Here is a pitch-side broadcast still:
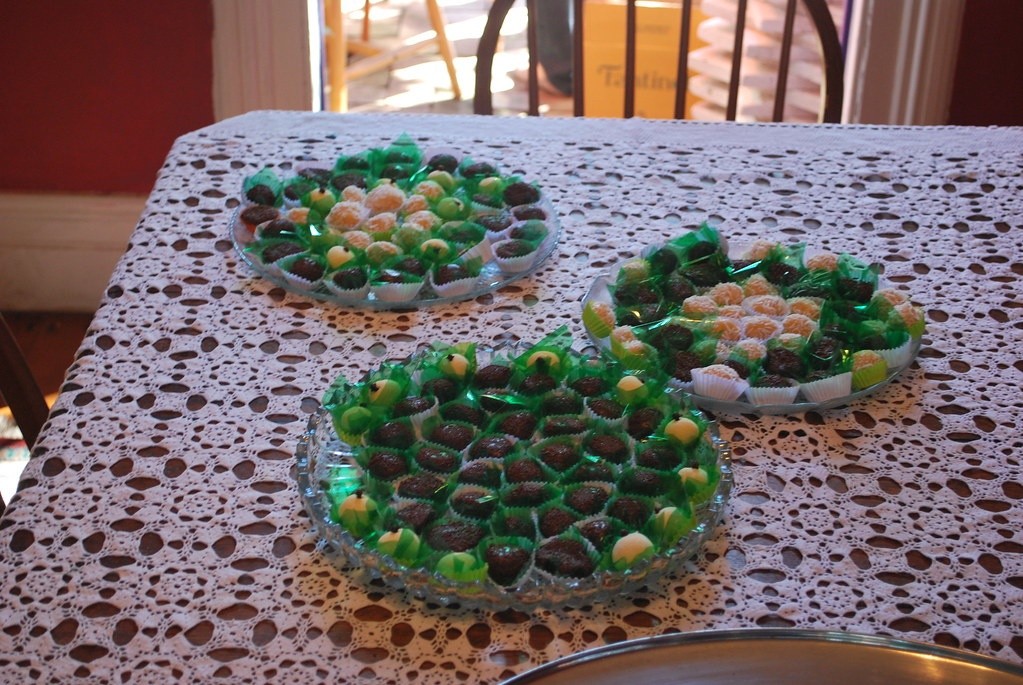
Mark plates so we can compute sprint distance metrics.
[492,626,1021,685]
[290,342,736,606]
[581,240,924,414]
[229,159,560,308]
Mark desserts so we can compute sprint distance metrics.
[583,222,927,407]
[315,321,725,593]
[238,134,553,303]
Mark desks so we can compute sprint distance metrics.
[2,108,1021,683]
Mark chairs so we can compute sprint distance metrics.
[472,0,844,122]
